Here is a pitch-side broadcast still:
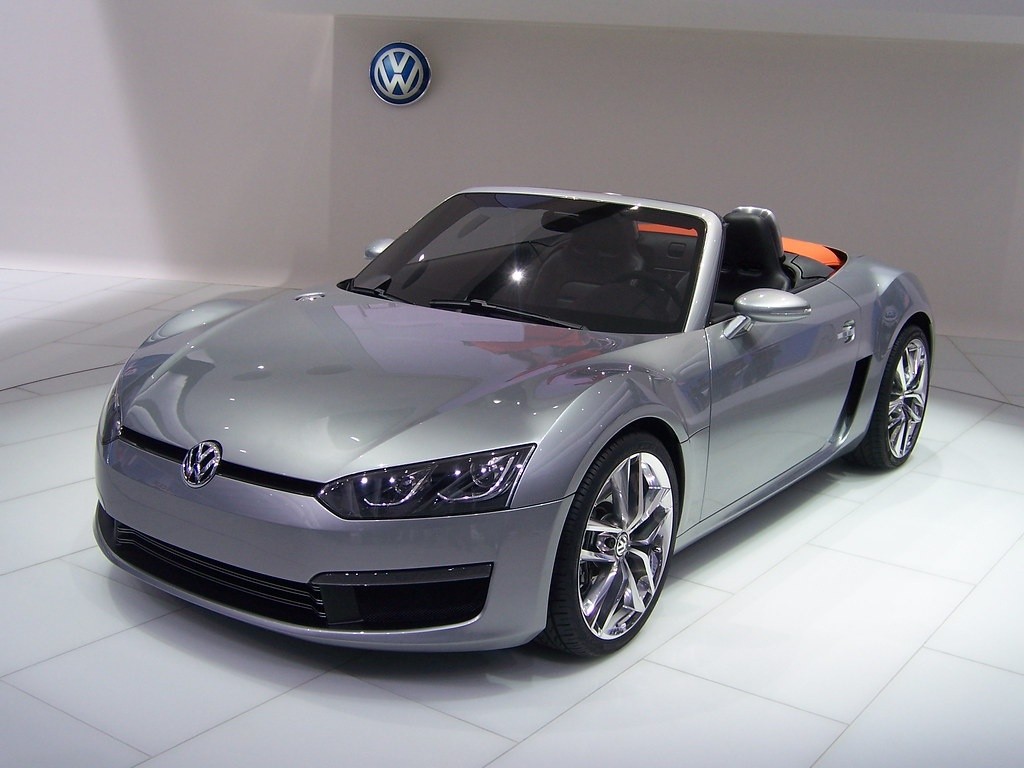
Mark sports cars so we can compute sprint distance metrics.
[93,186,935,660]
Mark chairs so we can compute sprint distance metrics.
[667,208,788,336]
[525,216,647,335]
